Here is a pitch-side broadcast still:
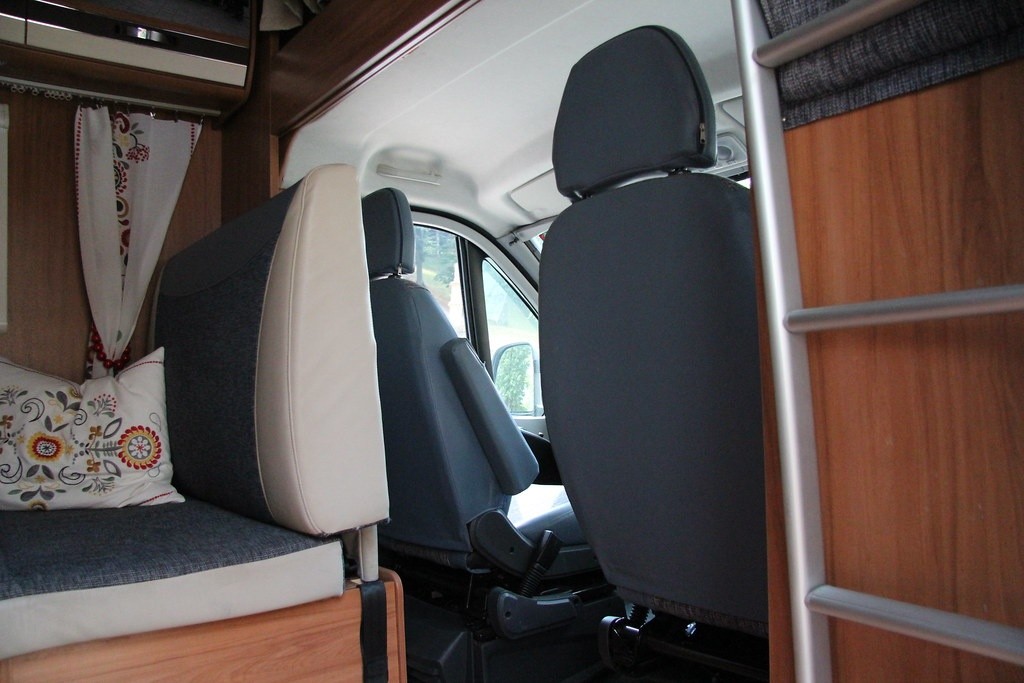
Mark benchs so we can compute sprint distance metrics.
[0,160,407,683]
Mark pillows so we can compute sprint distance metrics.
[0,345,187,510]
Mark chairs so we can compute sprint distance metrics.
[549,24,771,682]
[359,186,610,639]
